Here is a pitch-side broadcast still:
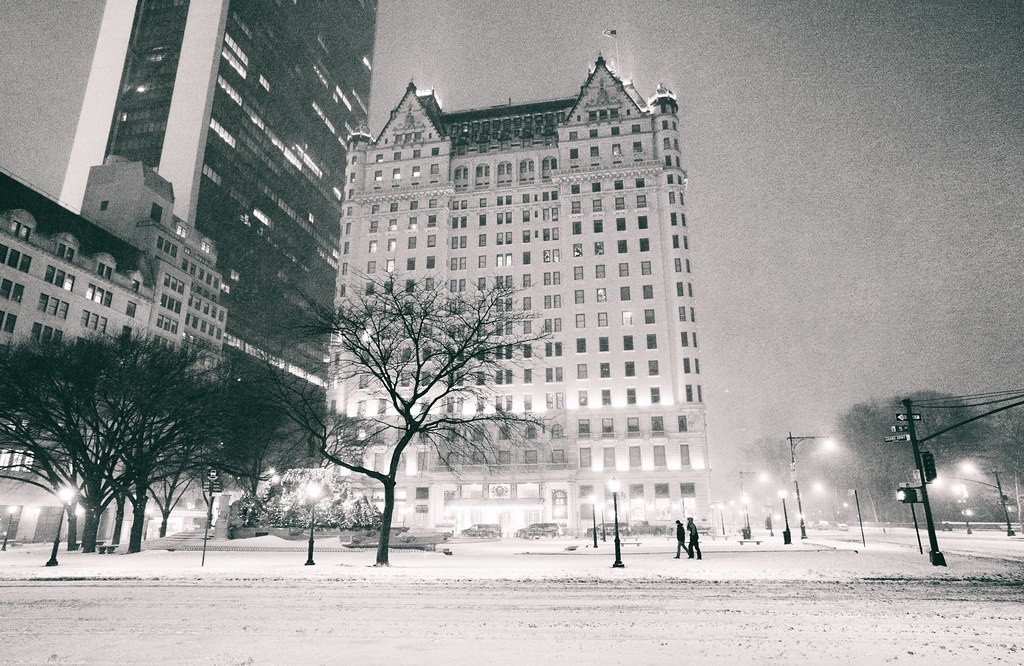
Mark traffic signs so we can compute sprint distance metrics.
[895,413,922,421]
[890,426,908,433]
[882,434,913,443]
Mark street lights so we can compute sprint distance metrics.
[142,509,153,542]
[787,433,835,539]
[303,483,321,566]
[719,502,727,536]
[608,479,625,569]
[779,490,792,539]
[739,470,768,534]
[1,505,18,552]
[45,487,74,567]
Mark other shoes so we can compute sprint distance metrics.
[674,556,680,559]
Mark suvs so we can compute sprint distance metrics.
[516,523,562,540]
[586,522,630,538]
[461,524,502,538]
[432,522,455,539]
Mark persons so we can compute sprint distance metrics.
[227,506,236,539]
[674,520,689,558]
[687,518,701,559]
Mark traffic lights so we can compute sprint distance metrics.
[922,452,938,481]
[897,489,917,503]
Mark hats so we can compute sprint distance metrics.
[687,517,693,521]
[676,520,680,523]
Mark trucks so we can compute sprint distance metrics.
[631,520,669,538]
